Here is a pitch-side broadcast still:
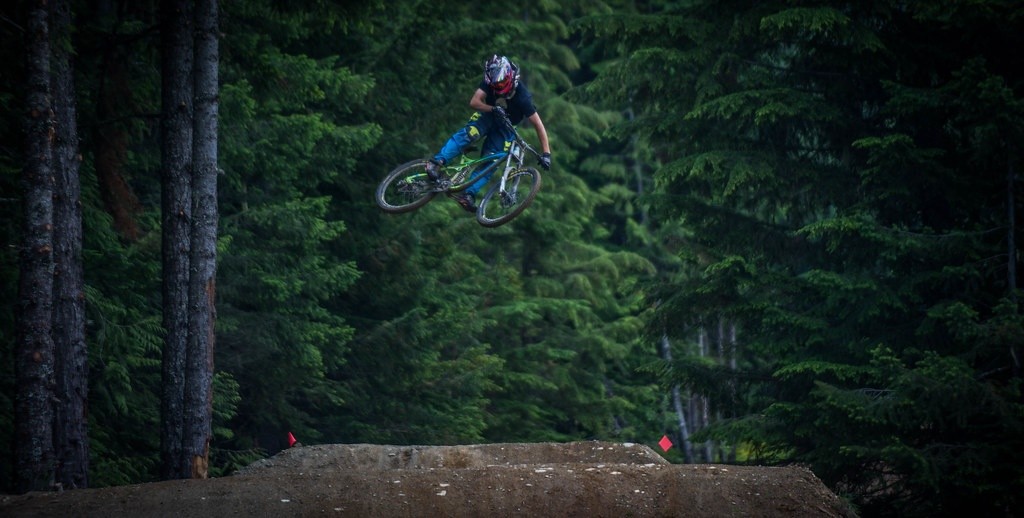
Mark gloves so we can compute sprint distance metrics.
[539,153,551,169]
[491,106,509,121]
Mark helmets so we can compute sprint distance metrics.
[484,54,521,98]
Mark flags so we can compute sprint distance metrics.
[658,435,672,452]
[289,432,296,447]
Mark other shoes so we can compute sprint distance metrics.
[461,193,478,212]
[425,159,442,180]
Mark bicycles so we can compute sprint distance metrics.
[375,108,549,226]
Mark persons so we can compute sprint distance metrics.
[425,54,550,212]
